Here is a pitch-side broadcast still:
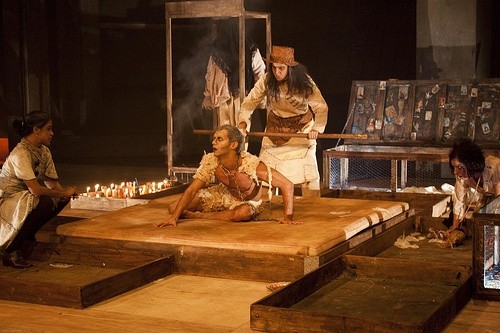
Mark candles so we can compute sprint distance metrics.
[86,178,171,199]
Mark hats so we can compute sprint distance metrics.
[262,45,299,66]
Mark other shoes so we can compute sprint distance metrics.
[3,249,32,269]
[23,239,50,260]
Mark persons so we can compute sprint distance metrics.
[236,45,328,198]
[152,125,295,230]
[202,18,266,152]
[445,141,500,237]
[0,111,81,268]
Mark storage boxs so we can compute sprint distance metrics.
[0,241,175,310]
[250,214,475,333]
